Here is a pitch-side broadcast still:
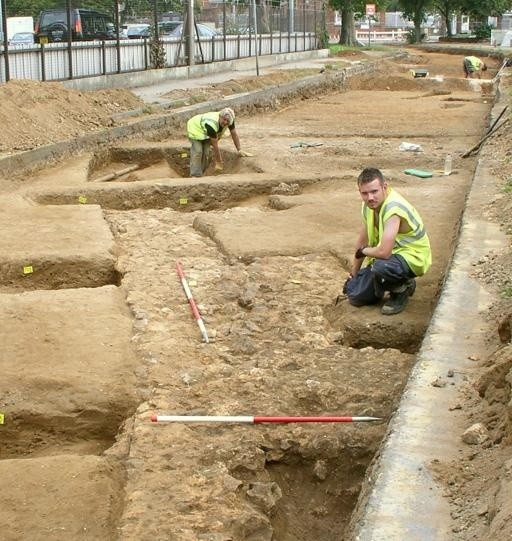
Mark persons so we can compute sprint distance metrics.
[463,56,487,79]
[342,167,432,315]
[186,107,253,177]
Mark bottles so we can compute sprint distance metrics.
[444,156,453,176]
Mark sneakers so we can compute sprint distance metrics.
[382,280,416,314]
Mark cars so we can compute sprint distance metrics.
[9,32,36,47]
[126,20,222,40]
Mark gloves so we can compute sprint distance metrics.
[343,273,353,294]
[215,161,224,174]
[239,149,253,156]
[355,246,366,259]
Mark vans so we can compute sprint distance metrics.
[34,7,123,46]
